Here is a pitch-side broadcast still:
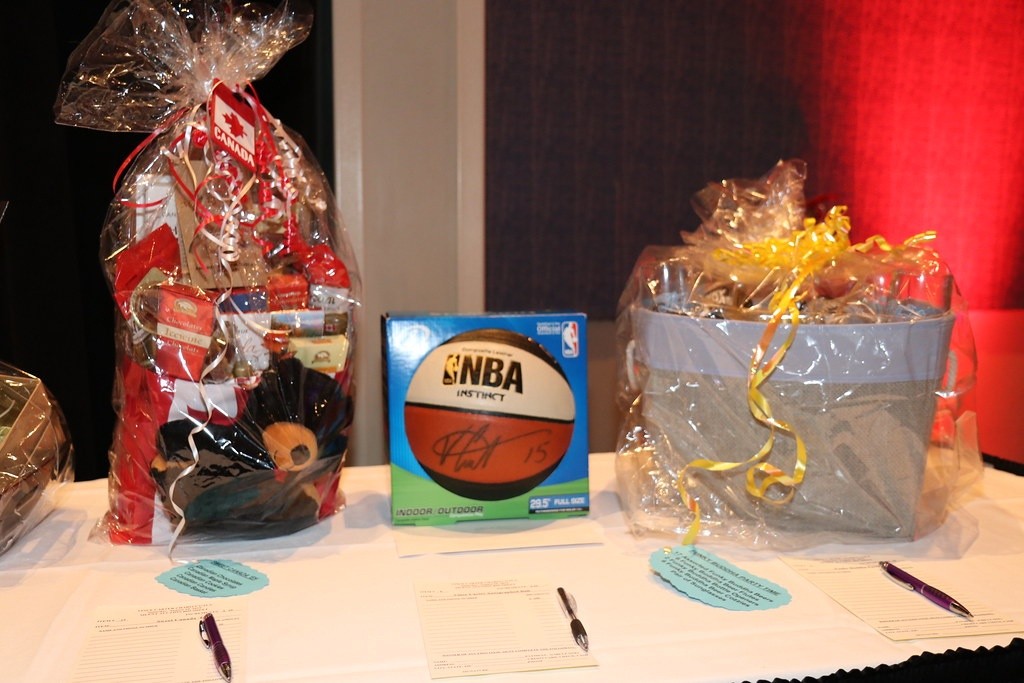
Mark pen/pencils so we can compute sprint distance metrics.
[557,587,589,650]
[198,613,231,683]
[879,561,973,618]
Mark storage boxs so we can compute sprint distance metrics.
[380,308,590,525]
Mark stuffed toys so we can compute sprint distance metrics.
[152,355,355,541]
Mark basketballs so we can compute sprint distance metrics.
[404,326,577,503]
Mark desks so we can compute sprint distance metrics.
[0,455,1024,683]
